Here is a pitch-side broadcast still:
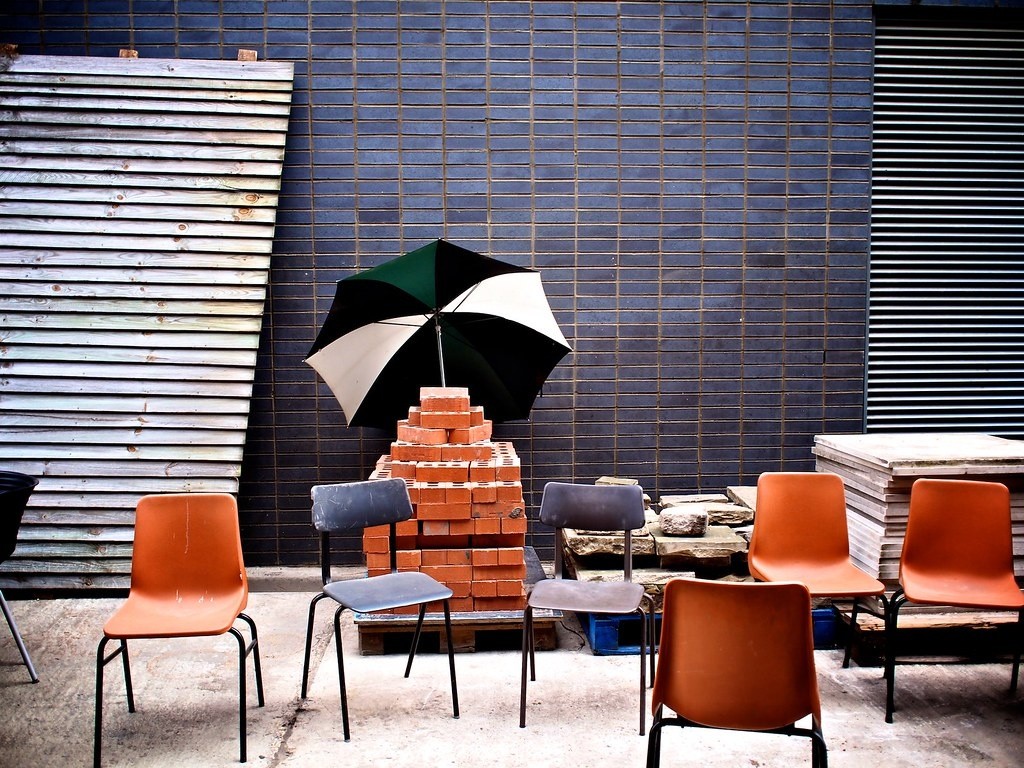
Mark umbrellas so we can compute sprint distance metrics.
[302,236,575,430]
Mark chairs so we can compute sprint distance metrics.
[301,476,459,743]
[647,576,827,767]
[0,471,39,682]
[94,494,265,768]
[518,481,655,736]
[883,478,1024,710]
[747,470,894,723]
